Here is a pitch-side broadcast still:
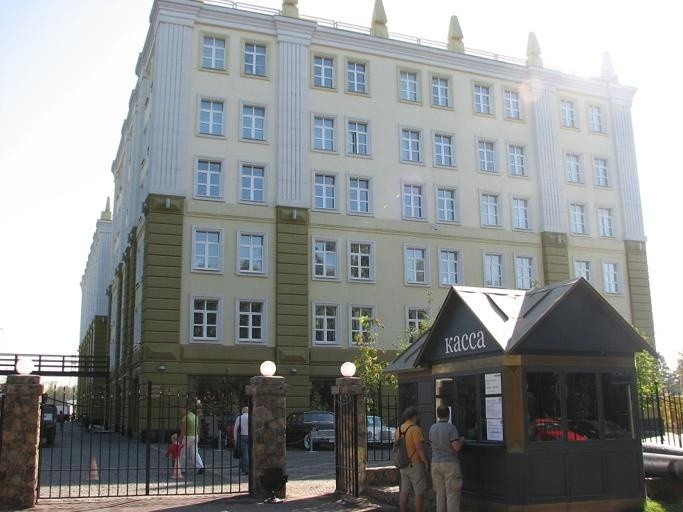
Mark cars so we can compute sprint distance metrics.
[366,415,396,449]
[286,410,336,451]
[528,415,631,442]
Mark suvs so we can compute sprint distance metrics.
[42,404,58,446]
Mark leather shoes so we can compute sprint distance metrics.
[196,467,206,475]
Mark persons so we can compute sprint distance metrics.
[232,406,250,475]
[390,404,430,512]
[179,404,208,474]
[165,432,184,472]
[428,404,466,512]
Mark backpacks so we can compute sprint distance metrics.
[392,424,419,470]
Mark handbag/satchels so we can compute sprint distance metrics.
[233,443,242,459]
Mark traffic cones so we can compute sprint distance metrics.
[170,455,183,478]
[86,454,100,481]
